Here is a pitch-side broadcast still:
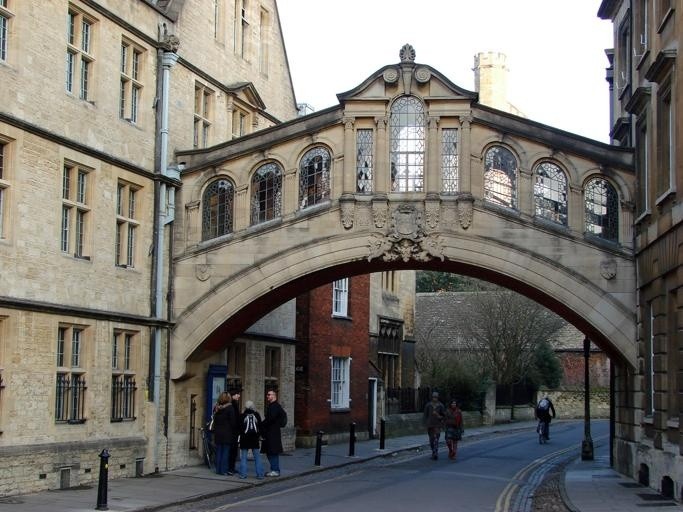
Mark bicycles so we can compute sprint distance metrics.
[534,415,554,444]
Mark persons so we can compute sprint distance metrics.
[213,392,235,476]
[535,393,556,440]
[239,401,264,479]
[215,387,240,473]
[259,388,283,476]
[433,399,466,460]
[422,391,445,460]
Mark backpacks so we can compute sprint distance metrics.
[243,413,259,435]
[538,399,549,410]
[279,407,288,428]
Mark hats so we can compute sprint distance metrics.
[431,392,438,397]
[541,392,548,399]
[227,387,244,395]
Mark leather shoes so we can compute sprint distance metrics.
[229,469,237,473]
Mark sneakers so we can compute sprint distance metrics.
[265,470,280,477]
[216,472,232,475]
